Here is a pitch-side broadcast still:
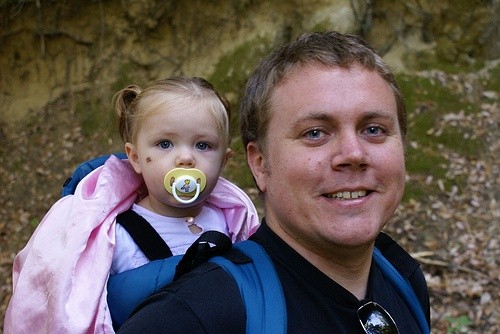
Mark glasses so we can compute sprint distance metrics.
[355,299,401,333]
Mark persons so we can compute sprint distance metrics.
[110,77,233,276]
[117,32,432,334]
